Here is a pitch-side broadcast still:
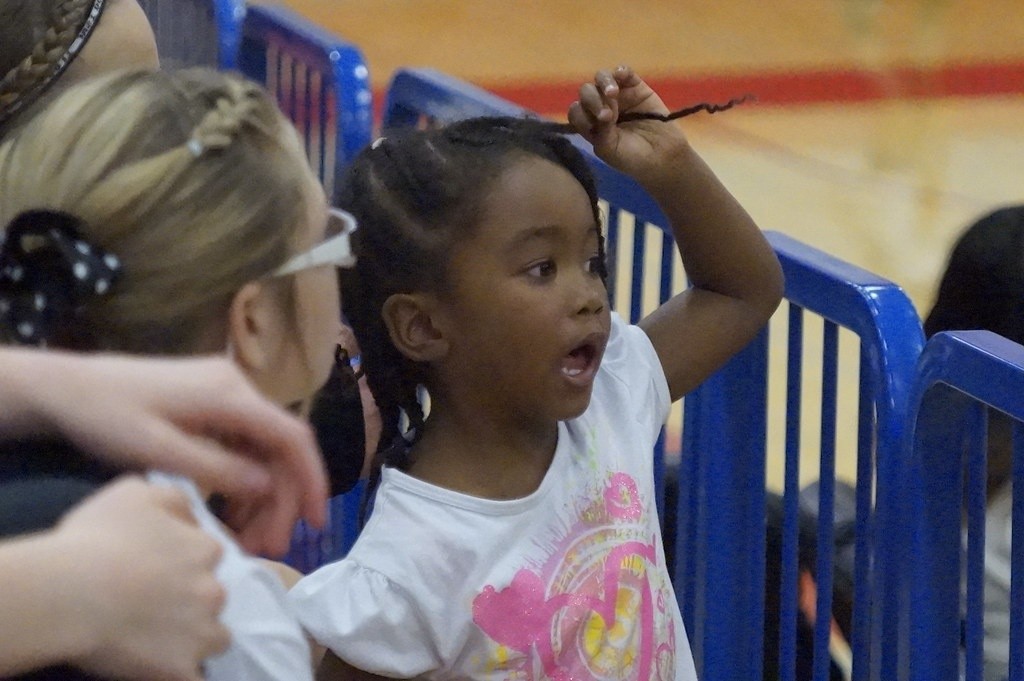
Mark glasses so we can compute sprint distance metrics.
[262,208,358,284]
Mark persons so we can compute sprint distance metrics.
[288,65,784,681]
[0,0,340,681]
[924,205,1024,681]
